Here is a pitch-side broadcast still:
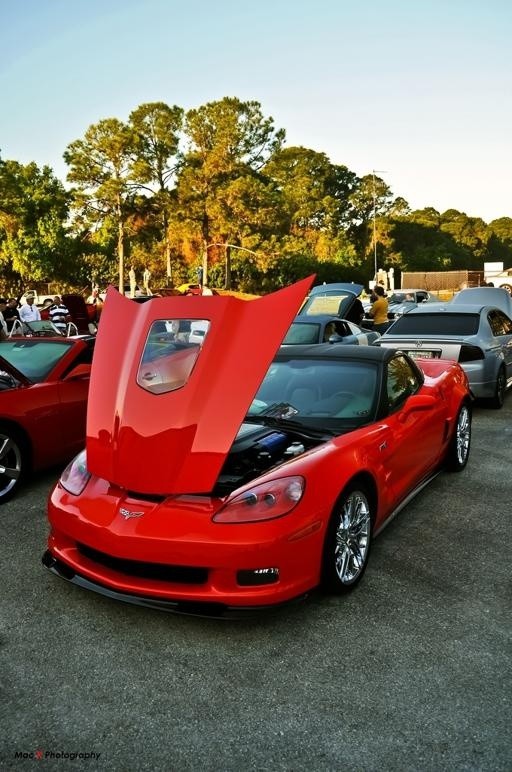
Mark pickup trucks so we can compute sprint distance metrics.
[19,290,63,307]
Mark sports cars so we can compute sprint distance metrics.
[44,273,470,616]
[0,318,98,505]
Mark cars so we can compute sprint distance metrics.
[85,283,220,304]
[40,302,97,323]
[359,287,512,405]
[280,280,380,347]
[485,268,512,294]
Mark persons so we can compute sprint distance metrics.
[367,285,391,336]
[194,265,203,290]
[0,282,104,341]
[401,293,415,302]
[143,266,154,297]
[337,292,366,328]
[129,264,138,297]
[369,285,387,302]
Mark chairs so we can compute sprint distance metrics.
[291,373,318,411]
